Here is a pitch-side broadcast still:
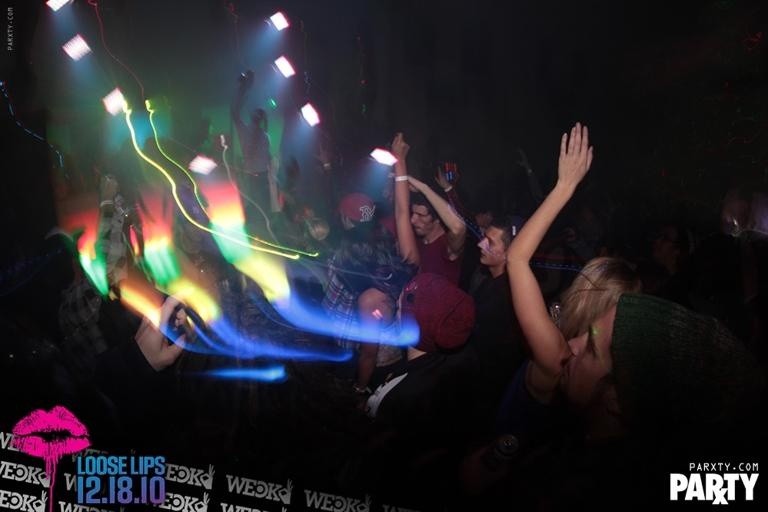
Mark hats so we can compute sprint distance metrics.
[608,290,768,447]
[401,272,476,353]
